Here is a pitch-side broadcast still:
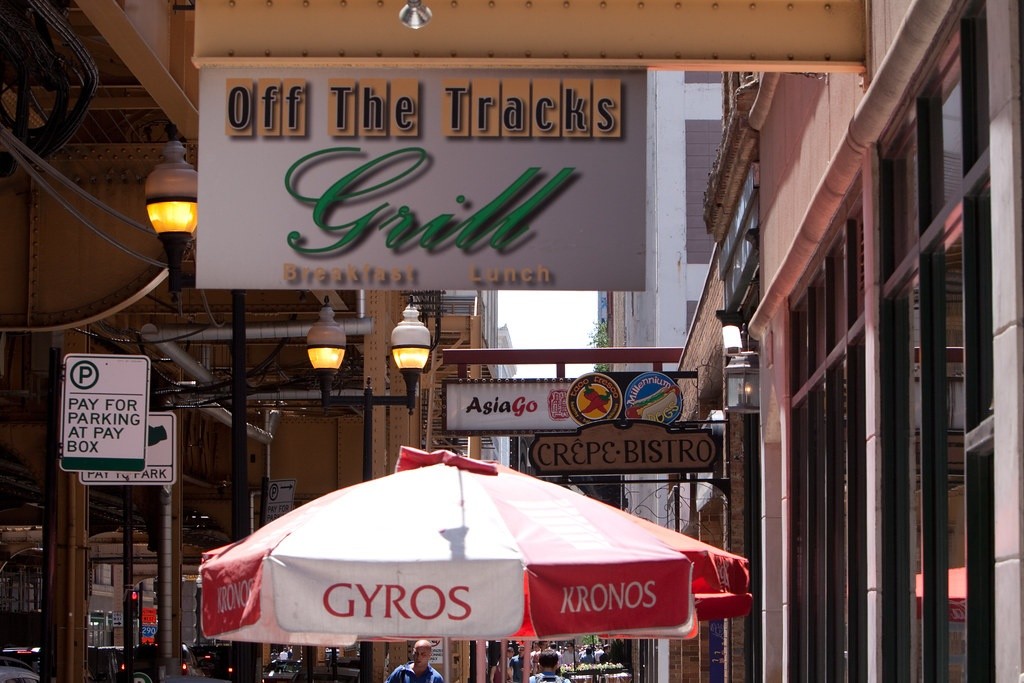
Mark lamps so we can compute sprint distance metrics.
[722,352,761,414]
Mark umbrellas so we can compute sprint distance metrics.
[199,445,754,683]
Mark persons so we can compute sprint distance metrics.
[386,640,444,683]
[486,641,612,683]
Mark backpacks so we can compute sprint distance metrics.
[534,673,565,683]
